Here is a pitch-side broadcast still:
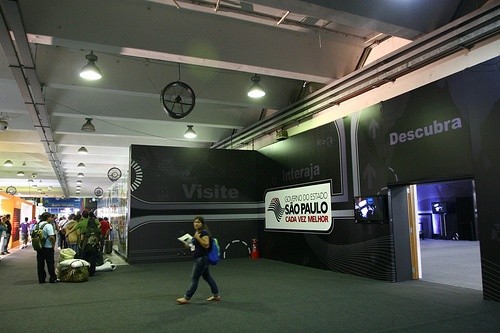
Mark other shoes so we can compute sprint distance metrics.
[177,298,189,304]
[207,296,221,302]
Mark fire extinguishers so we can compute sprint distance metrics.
[249,238,258,261]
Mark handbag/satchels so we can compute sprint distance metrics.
[58,260,89,283]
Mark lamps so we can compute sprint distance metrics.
[78,163,85,168]
[3,160,13,168]
[184,126,197,138]
[79,118,96,131]
[246,74,265,99]
[78,147,88,154]
[17,171,84,194]
[79,51,102,80]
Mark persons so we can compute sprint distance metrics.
[48,210,112,276]
[0,214,11,255]
[29,217,37,242]
[177,216,222,304]
[19,217,29,249]
[37,212,60,283]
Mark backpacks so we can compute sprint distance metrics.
[198,230,220,265]
[86,228,99,248]
[30,223,50,252]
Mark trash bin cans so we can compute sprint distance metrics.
[104,240,113,254]
